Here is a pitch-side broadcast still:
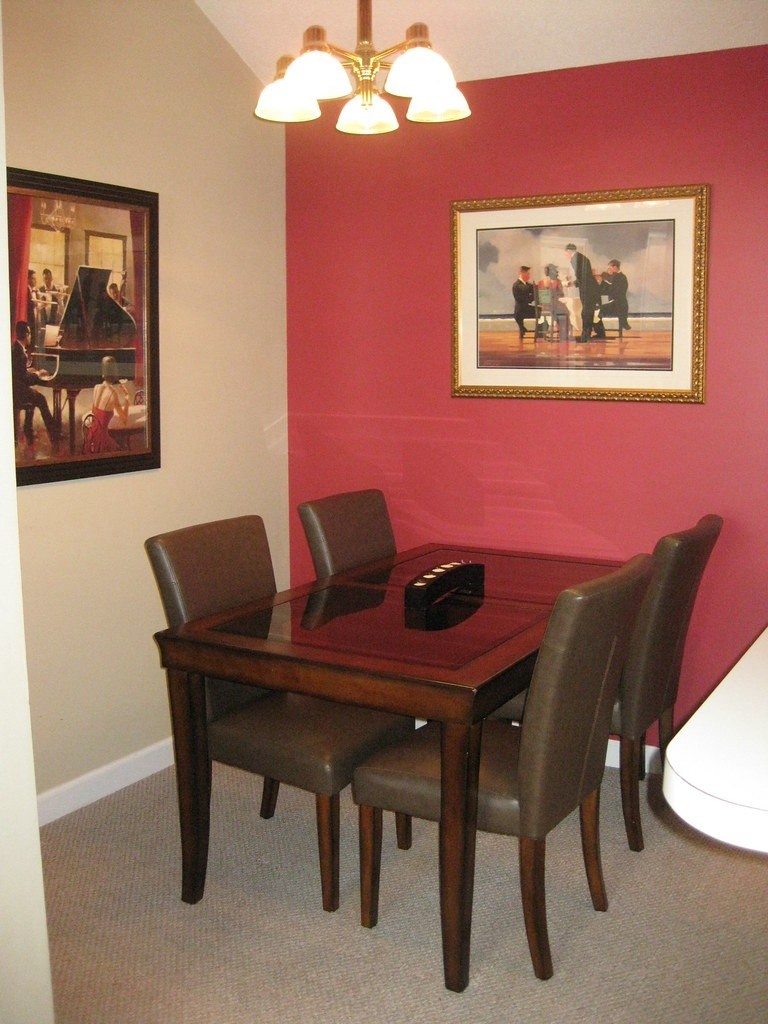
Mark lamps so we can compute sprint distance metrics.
[254,1,471,134]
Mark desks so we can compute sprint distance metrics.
[153,543,628,992]
[662,624,768,854]
[558,298,600,343]
[82,405,146,452]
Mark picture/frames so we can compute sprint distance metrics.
[449,183,707,404]
[7,167,160,486]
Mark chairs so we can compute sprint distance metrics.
[147,514,412,909]
[533,281,569,344]
[483,514,723,852]
[82,390,144,452]
[301,490,396,579]
[600,313,622,338]
[352,555,658,981]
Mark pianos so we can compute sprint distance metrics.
[31,265,140,454]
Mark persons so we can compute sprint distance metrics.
[10,268,138,454]
[512,243,628,343]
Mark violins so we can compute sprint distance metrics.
[594,272,613,283]
